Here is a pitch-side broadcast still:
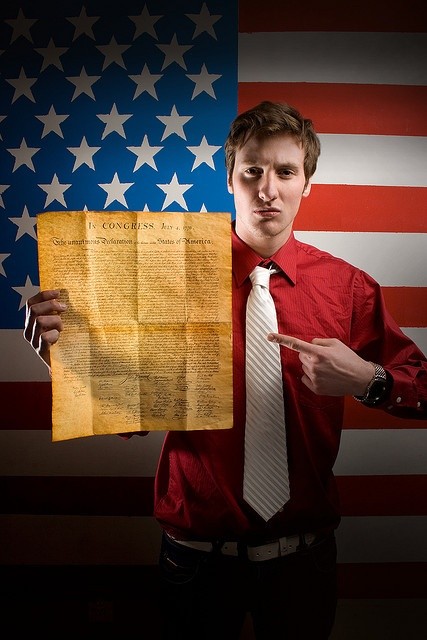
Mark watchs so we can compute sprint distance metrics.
[353,359,388,408]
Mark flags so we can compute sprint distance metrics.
[0,2,427,520]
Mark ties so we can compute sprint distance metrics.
[243,263,290,522]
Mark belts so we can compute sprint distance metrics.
[164,529,315,561]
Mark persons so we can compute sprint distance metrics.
[22,101,427,639]
